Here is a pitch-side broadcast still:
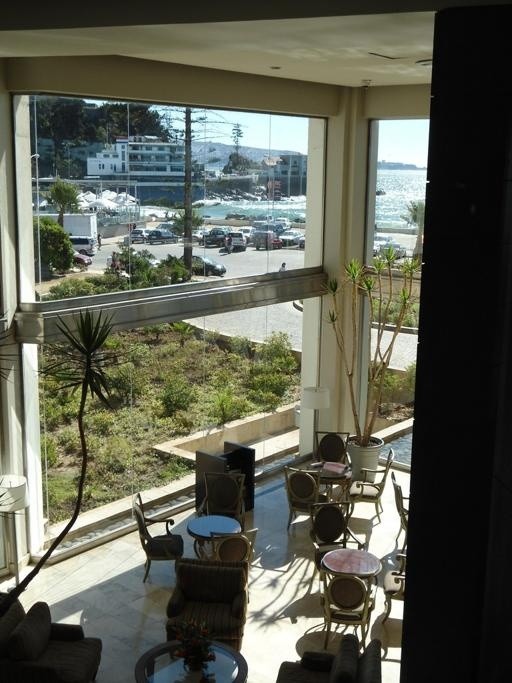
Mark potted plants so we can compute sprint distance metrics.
[320,245,422,482]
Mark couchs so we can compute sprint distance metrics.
[276,634,381,683]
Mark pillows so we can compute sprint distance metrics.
[329,634,358,683]
[10,602,52,661]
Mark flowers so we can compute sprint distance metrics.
[171,616,216,671]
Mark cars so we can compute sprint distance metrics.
[372,233,406,259]
[73,247,93,266]
[128,214,306,255]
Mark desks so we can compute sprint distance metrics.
[135,640,248,683]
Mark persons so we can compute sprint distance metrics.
[97,233,102,250]
[133,224,136,229]
[279,262,287,273]
[227,235,232,254]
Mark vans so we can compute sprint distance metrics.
[68,235,97,254]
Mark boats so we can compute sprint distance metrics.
[376,187,386,196]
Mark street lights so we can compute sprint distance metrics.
[31,152,41,161]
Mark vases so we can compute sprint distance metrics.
[185,663,202,683]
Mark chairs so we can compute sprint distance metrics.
[0,592,102,683]
